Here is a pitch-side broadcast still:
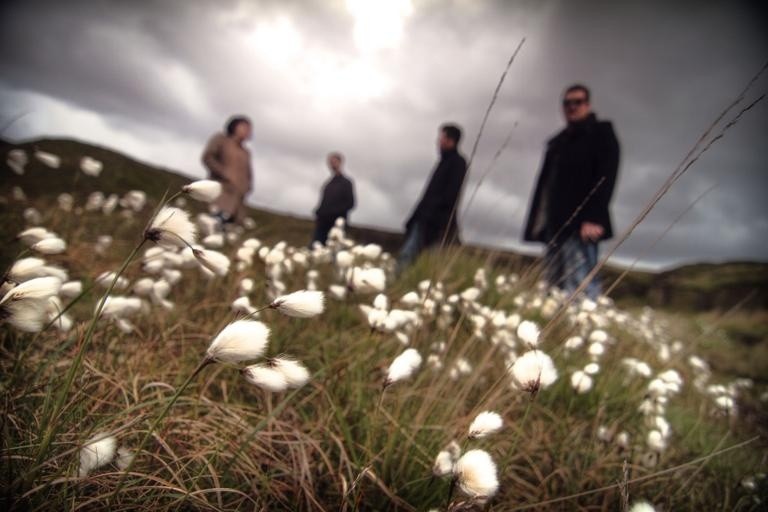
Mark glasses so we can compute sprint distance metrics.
[563,96,586,105]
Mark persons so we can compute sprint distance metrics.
[520,83,622,299]
[310,149,358,250]
[200,113,255,232]
[390,122,469,281]
